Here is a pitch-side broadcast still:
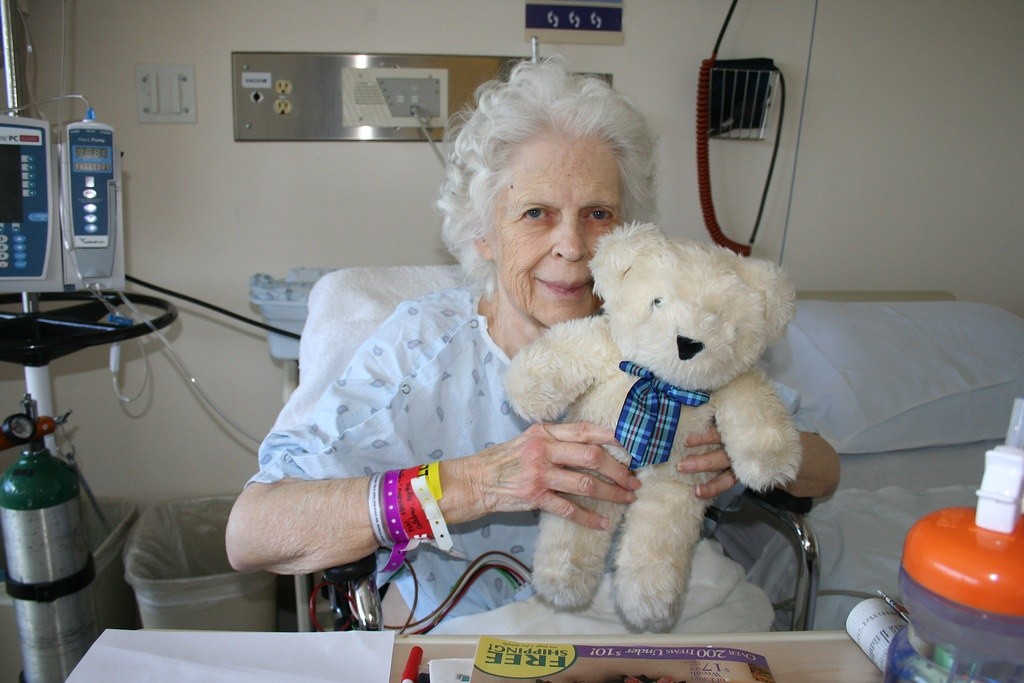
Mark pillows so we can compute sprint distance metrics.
[761,300,1024,454]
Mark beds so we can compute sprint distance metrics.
[704,290,1024,630]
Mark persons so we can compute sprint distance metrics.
[223,51,842,630]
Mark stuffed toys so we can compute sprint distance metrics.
[502,220,804,634]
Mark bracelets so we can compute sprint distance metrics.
[365,460,455,576]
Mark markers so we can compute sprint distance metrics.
[400,645,423,683]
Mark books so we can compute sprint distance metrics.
[427,634,775,683]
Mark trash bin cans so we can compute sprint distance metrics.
[0,495,136,683]
[125,493,278,633]
